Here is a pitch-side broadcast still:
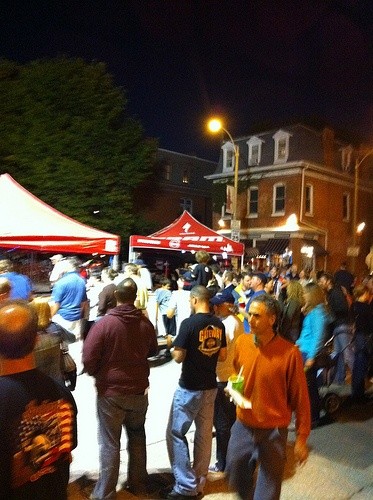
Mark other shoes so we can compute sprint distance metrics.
[161,488,199,500]
[209,463,223,473]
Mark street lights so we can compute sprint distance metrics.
[205,118,241,219]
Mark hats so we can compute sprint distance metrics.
[208,289,235,304]
[248,272,267,284]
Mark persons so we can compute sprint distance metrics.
[263,277,278,300]
[190,249,213,286]
[30,297,77,394]
[244,272,267,333]
[29,256,53,297]
[210,289,246,473]
[280,280,303,343]
[84,265,101,334]
[216,294,313,499]
[227,262,318,292]
[315,270,357,395]
[152,276,177,339]
[51,255,87,338]
[158,285,228,500]
[79,278,161,499]
[1,258,33,302]
[220,271,238,303]
[294,282,335,429]
[212,264,224,288]
[206,275,221,298]
[172,276,193,337]
[0,301,79,499]
[113,263,147,316]
[1,276,16,307]
[352,283,373,399]
[89,267,119,331]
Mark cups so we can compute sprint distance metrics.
[239,300,245,308]
[228,375,244,394]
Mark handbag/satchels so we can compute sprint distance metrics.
[56,323,76,392]
[313,352,331,368]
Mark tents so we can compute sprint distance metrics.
[129,208,244,256]
[0,172,122,256]
